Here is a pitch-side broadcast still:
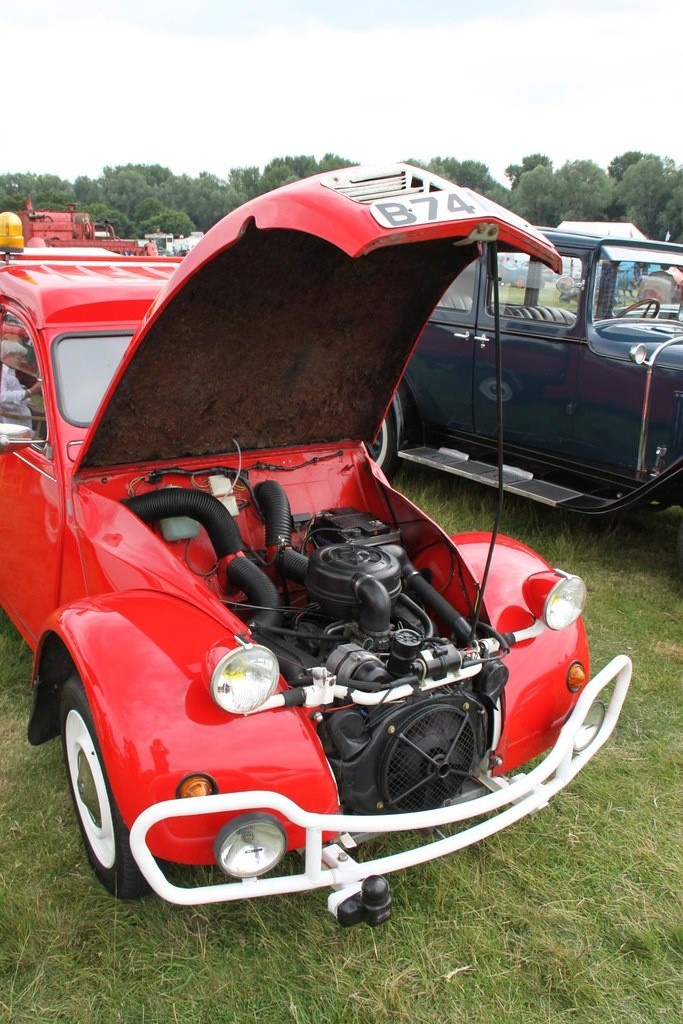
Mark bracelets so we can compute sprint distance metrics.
[25,389,32,398]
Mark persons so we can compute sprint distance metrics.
[0,338,47,444]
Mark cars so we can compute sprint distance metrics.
[365,227,683,516]
[94,228,209,261]
[0,159,633,931]
[490,252,682,318]
